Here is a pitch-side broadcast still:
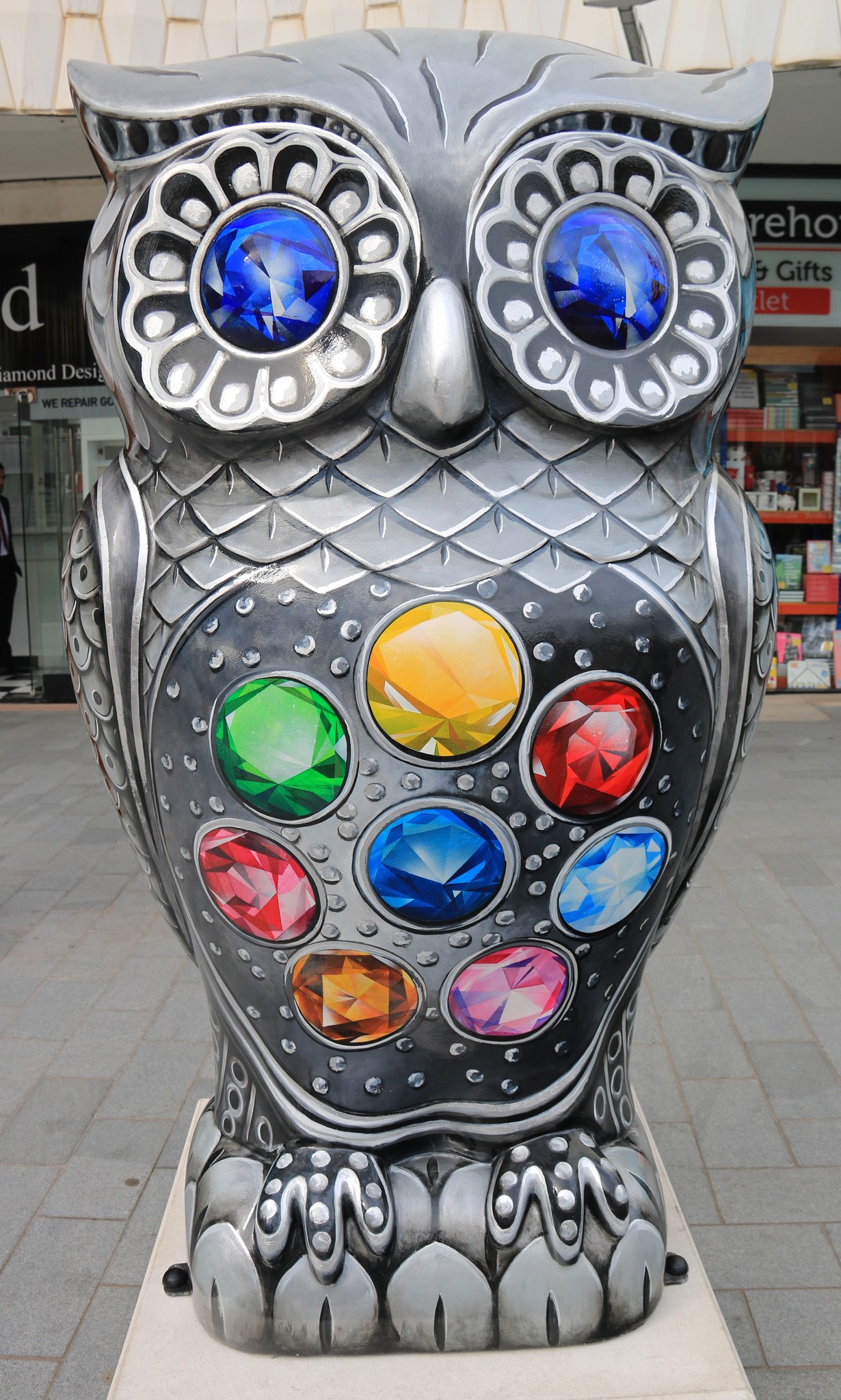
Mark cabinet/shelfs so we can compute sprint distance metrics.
[724,429,840,616]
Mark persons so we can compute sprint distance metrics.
[0,462,24,671]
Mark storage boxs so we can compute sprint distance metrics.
[802,572,838,603]
